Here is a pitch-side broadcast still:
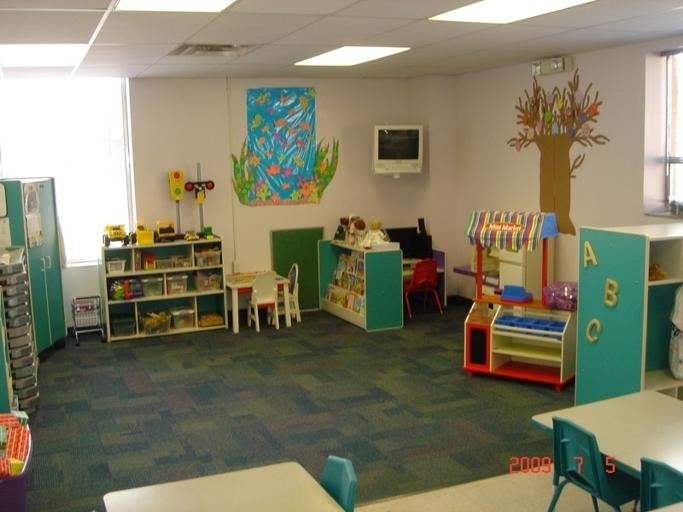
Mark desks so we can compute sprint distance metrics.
[103,459,344,511]
[528,388,682,482]
[224,273,293,333]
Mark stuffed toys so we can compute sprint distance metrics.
[335,214,390,251]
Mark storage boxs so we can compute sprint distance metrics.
[140,310,171,333]
[169,306,194,330]
[140,276,162,296]
[110,313,135,334]
[106,256,126,272]
[167,273,186,291]
[192,272,221,290]
[193,250,221,265]
[155,257,173,268]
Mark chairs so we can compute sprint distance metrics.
[638,455,682,512]
[320,453,353,510]
[267,262,305,325]
[244,269,279,332]
[545,414,636,512]
[405,259,448,316]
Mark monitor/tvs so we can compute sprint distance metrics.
[372,124,424,175]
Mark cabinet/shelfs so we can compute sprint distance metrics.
[316,238,404,332]
[461,209,578,391]
[0,176,68,357]
[571,219,683,407]
[99,230,228,344]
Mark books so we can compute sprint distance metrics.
[320,251,364,317]
